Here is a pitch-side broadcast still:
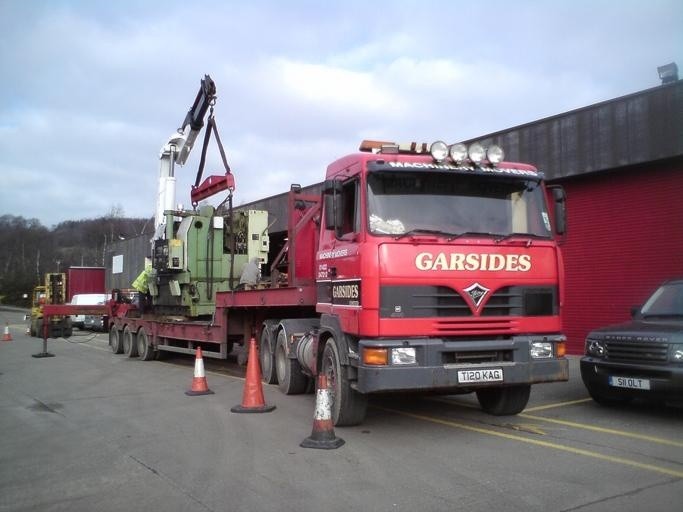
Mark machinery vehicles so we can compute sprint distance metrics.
[30,272,72,338]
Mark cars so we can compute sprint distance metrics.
[580,279,683,405]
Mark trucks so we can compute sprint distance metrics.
[32,74,569,426]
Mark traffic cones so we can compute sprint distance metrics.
[0,321,12,341]
[185,347,214,396]
[231,338,276,412]
[299,374,345,449]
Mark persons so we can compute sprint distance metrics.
[131,266,152,316]
[238,256,261,285]
